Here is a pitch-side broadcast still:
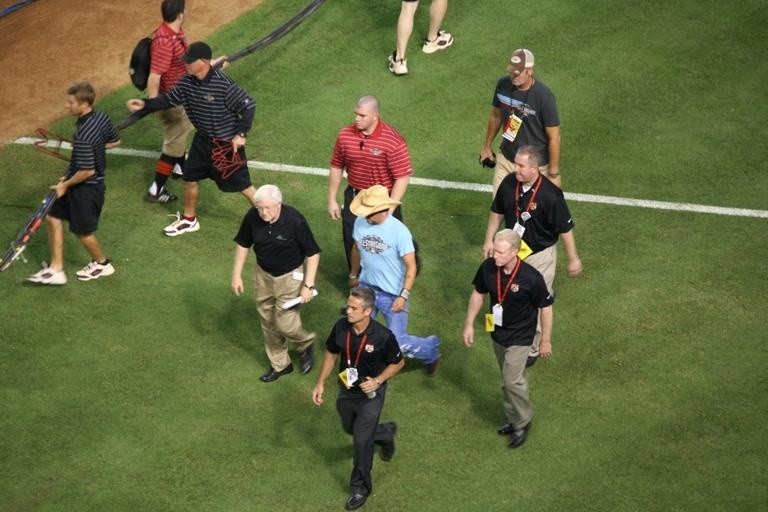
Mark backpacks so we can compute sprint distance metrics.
[128,26,176,93]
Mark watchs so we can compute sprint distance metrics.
[235,132,243,136]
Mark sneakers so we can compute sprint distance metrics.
[75,260,115,281]
[422,31,454,54]
[163,211,200,237]
[24,268,68,285]
[144,188,178,203]
[387,50,408,76]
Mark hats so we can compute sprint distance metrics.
[180,42,212,64]
[504,49,534,75]
[349,184,403,218]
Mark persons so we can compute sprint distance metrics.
[311,284,406,511]
[384,0,455,76]
[142,0,227,205]
[125,42,256,237]
[24,82,120,286]
[230,183,323,383]
[342,184,442,376]
[484,145,582,367]
[462,228,553,449]
[479,49,561,201]
[329,96,419,277]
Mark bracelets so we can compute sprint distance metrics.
[547,172,559,178]
[400,287,409,299]
[372,375,383,383]
[302,281,315,289]
[349,272,357,277]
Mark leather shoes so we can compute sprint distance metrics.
[526,353,541,368]
[298,345,313,375]
[425,358,439,376]
[259,363,293,382]
[346,488,373,509]
[381,422,397,461]
[507,421,530,448]
[496,422,512,436]
